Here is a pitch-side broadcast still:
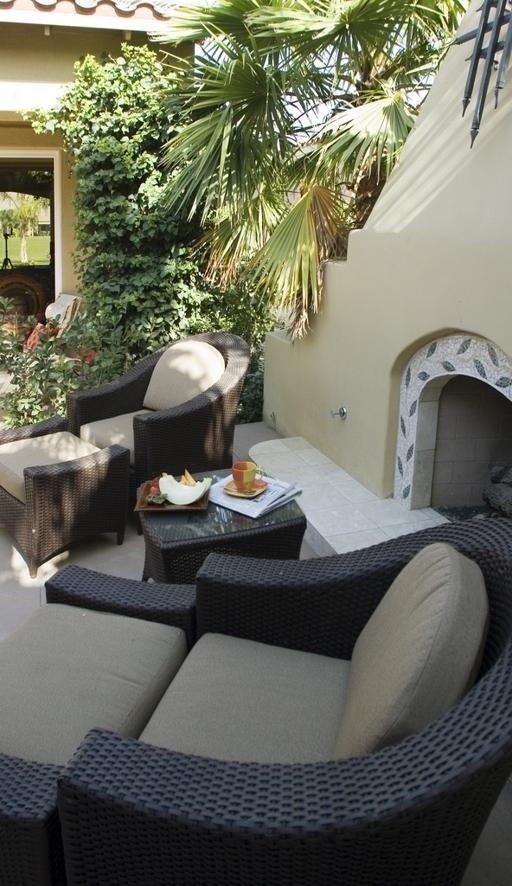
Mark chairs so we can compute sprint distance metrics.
[71,332,248,536]
[57,514,512,882]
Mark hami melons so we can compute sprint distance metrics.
[158,473,212,506]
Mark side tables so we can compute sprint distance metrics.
[130,457,310,588]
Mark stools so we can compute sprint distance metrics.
[0,415,132,581]
[0,561,198,881]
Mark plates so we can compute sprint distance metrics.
[132,479,212,514]
[222,477,268,499]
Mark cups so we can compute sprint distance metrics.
[231,461,263,494]
